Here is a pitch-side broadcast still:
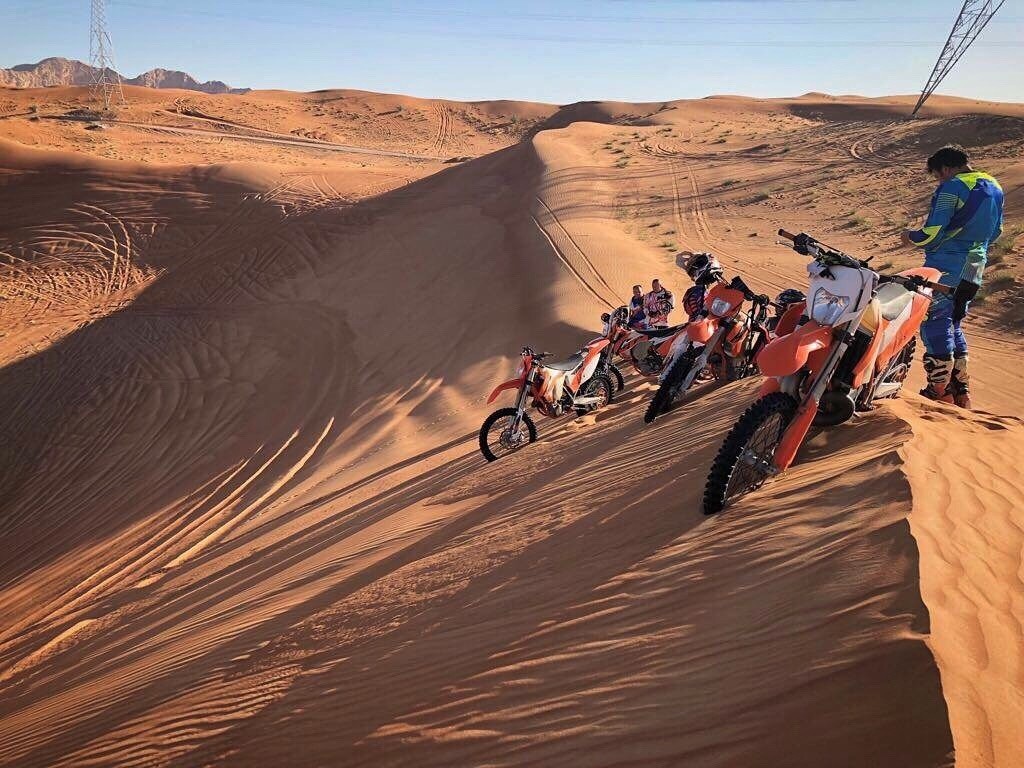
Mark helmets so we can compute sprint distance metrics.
[686,253,723,286]
[774,289,807,317]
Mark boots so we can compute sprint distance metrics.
[950,353,971,409]
[919,352,955,404]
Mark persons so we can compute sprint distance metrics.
[683,283,708,322]
[628,279,674,329]
[901,145,1004,409]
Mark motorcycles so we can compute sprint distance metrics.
[479,336,611,462]
[595,272,784,424]
[702,228,959,514]
